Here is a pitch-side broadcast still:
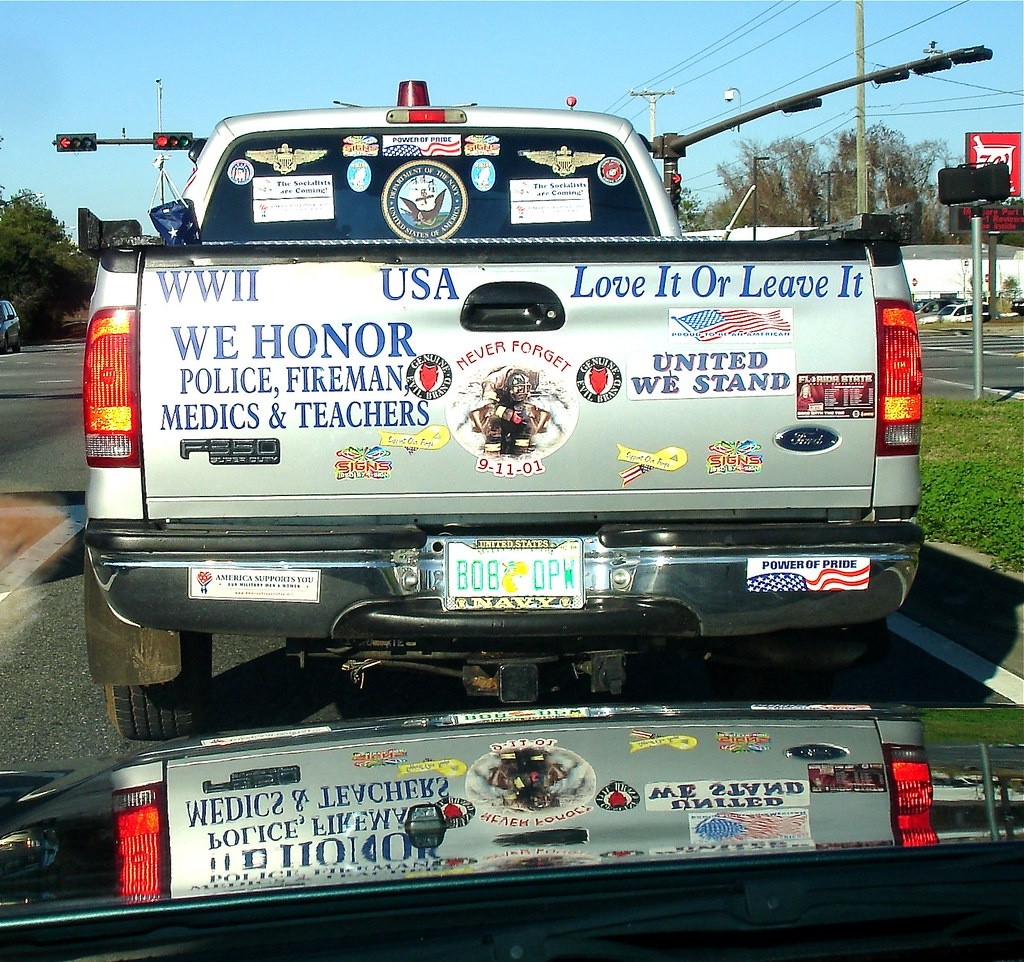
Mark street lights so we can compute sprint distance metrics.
[821,169,836,225]
[753,157,769,241]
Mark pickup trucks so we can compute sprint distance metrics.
[79,80,925,741]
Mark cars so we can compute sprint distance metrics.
[914,293,1024,324]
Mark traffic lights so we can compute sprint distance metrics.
[57,133,96,151]
[154,132,192,150]
[670,173,682,205]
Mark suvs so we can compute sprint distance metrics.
[0,300,21,354]
